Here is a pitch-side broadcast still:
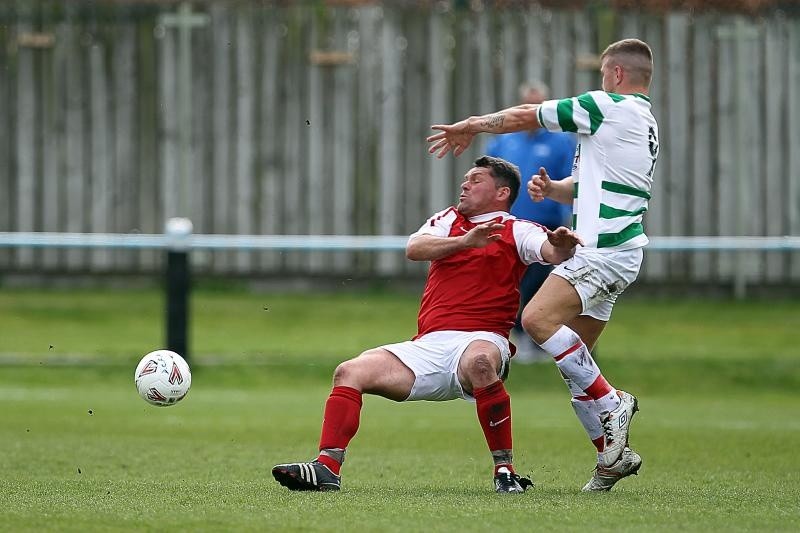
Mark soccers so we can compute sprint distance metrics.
[133,348,194,408]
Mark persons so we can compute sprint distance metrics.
[271,152,585,493]
[483,75,583,366]
[426,37,664,493]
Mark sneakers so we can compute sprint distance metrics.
[598,390,639,468]
[581,444,642,492]
[494,473,535,493]
[271,458,341,491]
